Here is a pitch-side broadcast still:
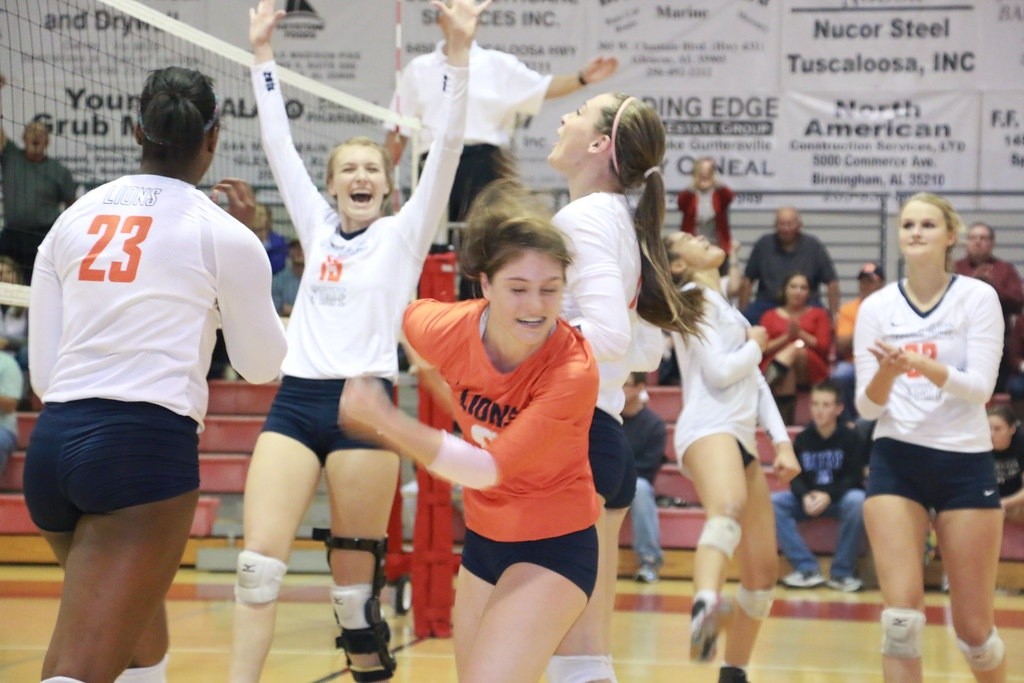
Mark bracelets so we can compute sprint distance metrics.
[578,70,586,86]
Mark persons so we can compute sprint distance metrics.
[382,0,618,302]
[981,404,1024,509]
[678,157,734,276]
[663,232,802,683]
[741,208,884,426]
[339,181,601,683]
[953,221,1024,403]
[230,0,493,682]
[771,379,866,592]
[0,74,305,482]
[22,66,289,683]
[546,93,717,583]
[853,191,1006,682]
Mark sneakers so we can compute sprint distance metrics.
[687,596,732,665]
[781,569,825,589]
[634,563,659,586]
[827,575,866,594]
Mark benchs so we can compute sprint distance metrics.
[1,380,1023,567]
